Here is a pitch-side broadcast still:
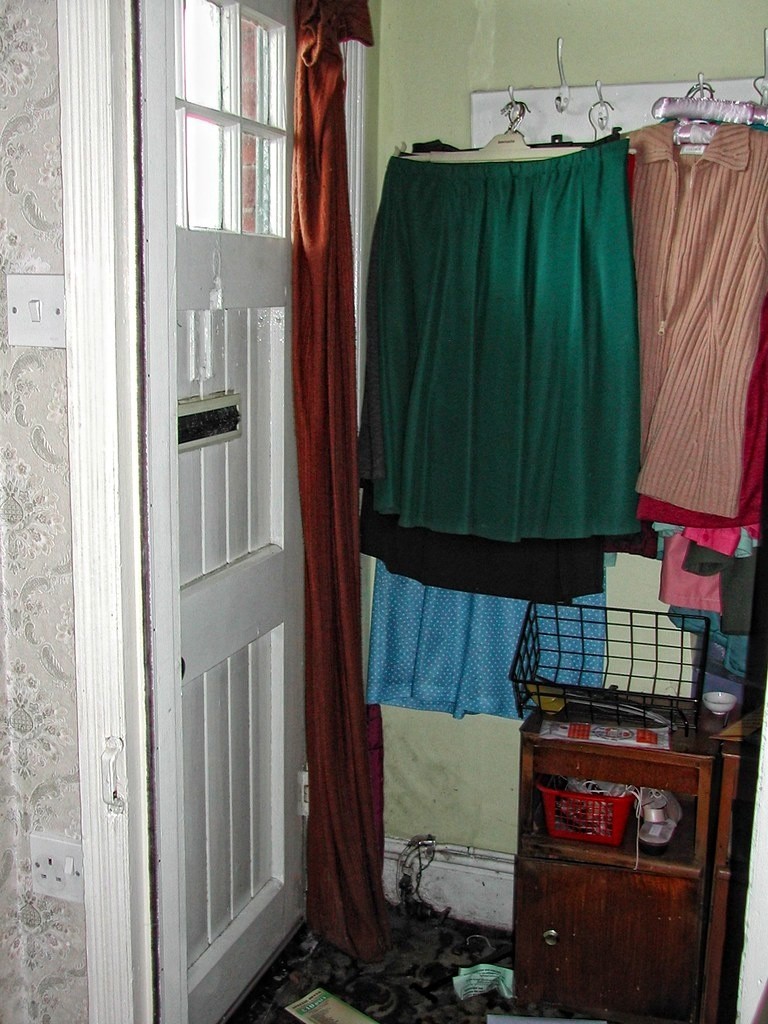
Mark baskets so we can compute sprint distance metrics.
[508,600,709,736]
[535,773,633,847]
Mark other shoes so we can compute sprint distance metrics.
[638,787,681,855]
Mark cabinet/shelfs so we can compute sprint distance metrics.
[512,696,744,1020]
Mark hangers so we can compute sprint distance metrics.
[393,78,768,176]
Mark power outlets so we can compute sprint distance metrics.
[31,835,85,904]
